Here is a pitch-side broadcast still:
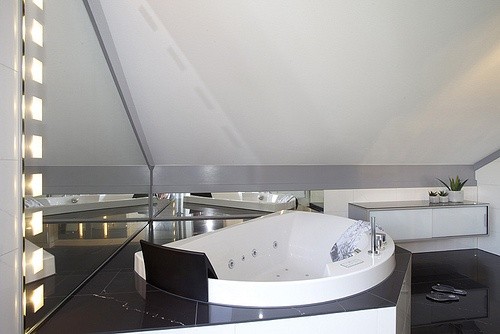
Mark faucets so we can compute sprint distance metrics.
[365,216,379,256]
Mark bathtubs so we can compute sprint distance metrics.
[153,213,395,283]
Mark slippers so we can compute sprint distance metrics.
[432,284,468,296]
[426,292,460,302]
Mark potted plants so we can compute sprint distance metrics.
[435,175,469,202]
[438,190,449,202]
[428,191,438,204]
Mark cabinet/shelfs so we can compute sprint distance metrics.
[348,202,489,240]
